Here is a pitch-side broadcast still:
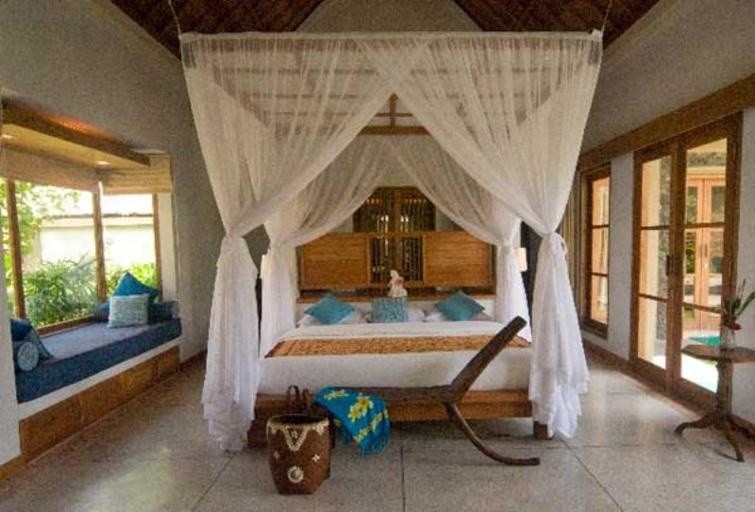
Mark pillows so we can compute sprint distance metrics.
[91,271,179,328]
[303,288,487,325]
[9,316,55,373]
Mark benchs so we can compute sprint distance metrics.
[20,317,181,405]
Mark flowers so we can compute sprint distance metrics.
[715,277,755,330]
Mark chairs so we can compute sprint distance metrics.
[310,317,545,467]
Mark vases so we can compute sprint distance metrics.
[720,327,736,349]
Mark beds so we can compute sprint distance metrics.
[218,290,550,451]
